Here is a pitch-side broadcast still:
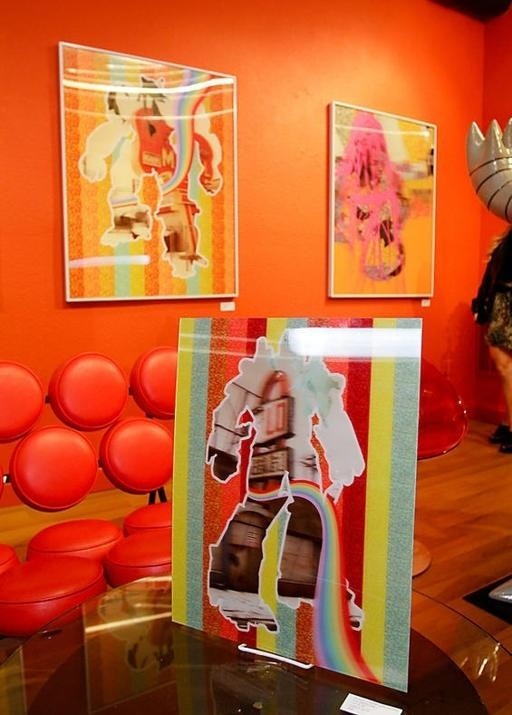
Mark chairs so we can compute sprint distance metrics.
[411,358,467,578]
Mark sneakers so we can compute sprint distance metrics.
[489,424,511,453]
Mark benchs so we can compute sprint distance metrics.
[0,345,178,638]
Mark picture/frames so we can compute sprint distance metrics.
[327,100,437,299]
[58,41,240,303]
